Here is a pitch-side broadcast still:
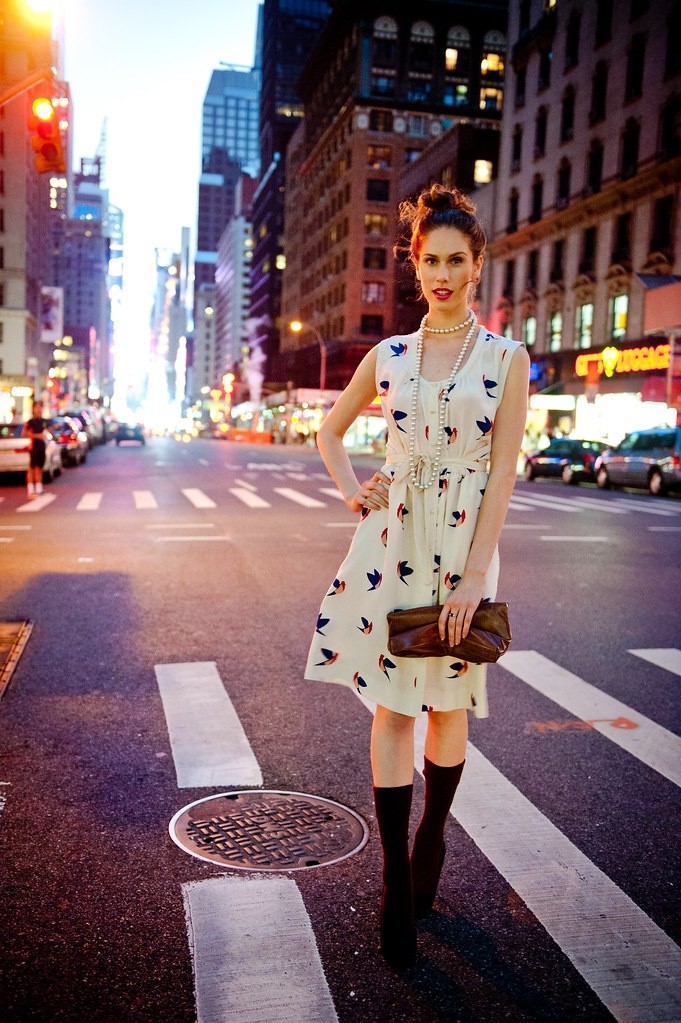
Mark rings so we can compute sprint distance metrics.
[449,613,457,617]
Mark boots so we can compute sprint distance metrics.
[372,784,417,974]
[410,755,465,919]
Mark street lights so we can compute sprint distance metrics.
[291,320,326,389]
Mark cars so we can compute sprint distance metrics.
[525,439,612,487]
[48,417,87,468]
[0,422,62,486]
[60,407,118,451]
[114,422,145,448]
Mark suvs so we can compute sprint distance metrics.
[594,428,681,496]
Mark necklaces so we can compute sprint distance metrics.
[409,307,477,490]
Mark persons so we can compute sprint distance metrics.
[21,400,53,499]
[304,183,531,983]
